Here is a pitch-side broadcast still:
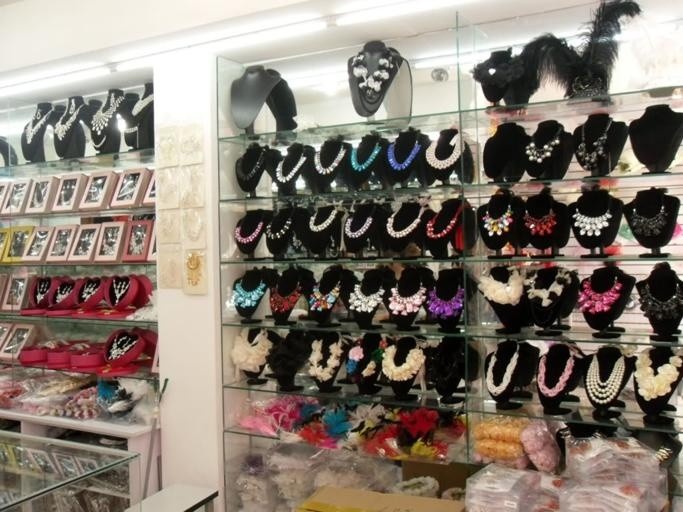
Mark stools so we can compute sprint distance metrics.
[128,484,219,510]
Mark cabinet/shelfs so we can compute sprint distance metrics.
[2,67,161,499]
[216,3,681,511]
[2,430,142,511]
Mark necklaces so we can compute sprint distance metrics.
[572,206,613,237]
[634,347,683,402]
[577,276,623,315]
[631,205,669,237]
[576,119,614,170]
[638,279,683,322]
[585,353,626,404]
